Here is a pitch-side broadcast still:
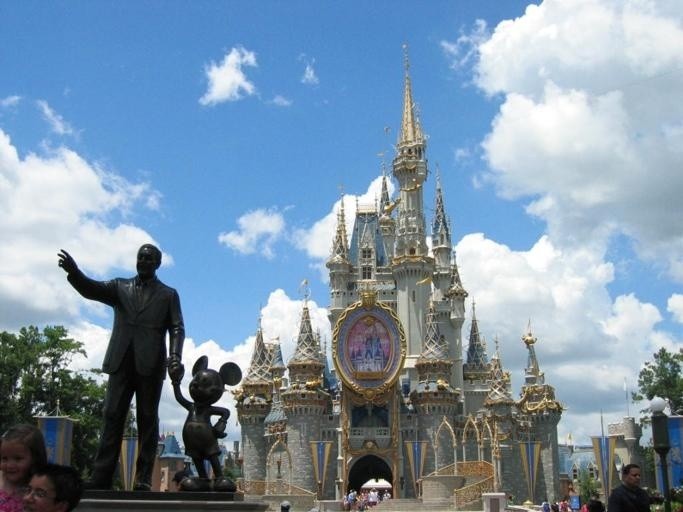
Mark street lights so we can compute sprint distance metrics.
[649,396,672,512]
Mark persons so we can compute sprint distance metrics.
[172,472,187,488]
[343,488,392,511]
[56,243,183,490]
[606,463,652,512]
[0,422,46,512]
[22,461,83,512]
[539,490,604,512]
